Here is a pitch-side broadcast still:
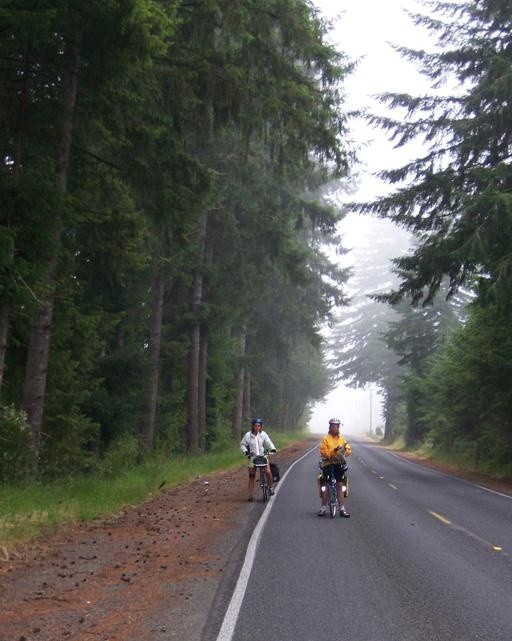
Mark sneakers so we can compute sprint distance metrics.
[248,496,254,502]
[340,510,350,518]
[317,510,326,517]
[269,486,275,496]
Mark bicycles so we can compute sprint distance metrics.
[318,458,348,517]
[247,450,274,503]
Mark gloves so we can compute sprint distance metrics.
[246,451,250,455]
[270,449,277,453]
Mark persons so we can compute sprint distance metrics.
[239,417,279,503]
[316,416,353,519]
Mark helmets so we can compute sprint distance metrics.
[329,418,341,425]
[251,417,263,425]
